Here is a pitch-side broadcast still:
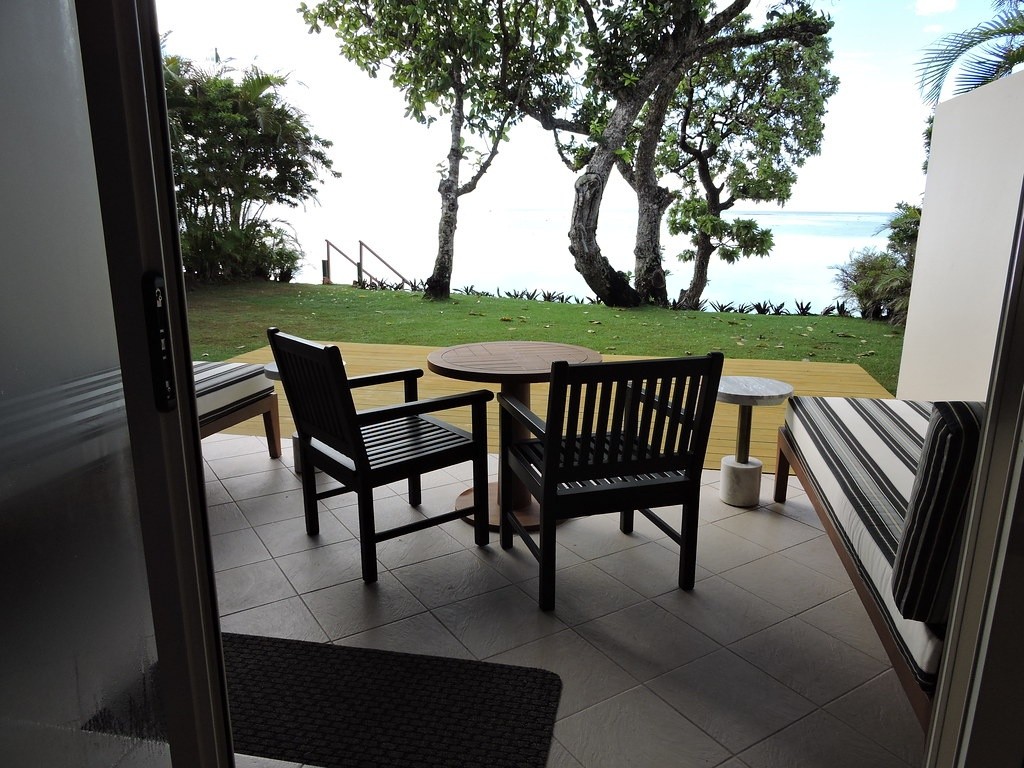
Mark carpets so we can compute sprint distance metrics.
[81,630,563,768]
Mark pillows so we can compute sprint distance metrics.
[890,401,987,625]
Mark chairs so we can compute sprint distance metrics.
[266,326,494,585]
[496,352,724,612]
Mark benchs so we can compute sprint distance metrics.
[773,394,945,752]
[0,361,288,510]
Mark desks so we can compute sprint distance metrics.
[716,376,794,508]
[428,341,603,534]
